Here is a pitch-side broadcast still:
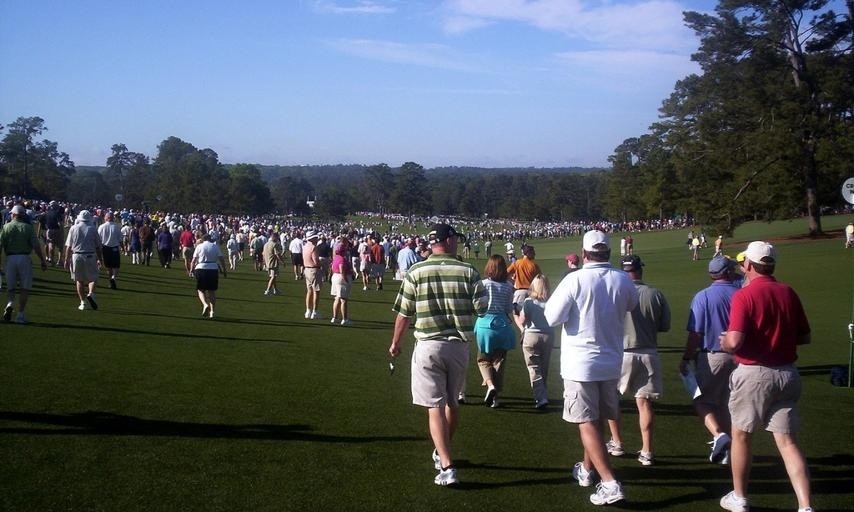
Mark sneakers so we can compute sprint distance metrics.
[604,438,624,458]
[353,274,385,292]
[305,310,354,326]
[46,257,63,266]
[719,493,746,512]
[589,479,625,506]
[295,273,304,282]
[78,295,96,311]
[456,386,549,412]
[573,459,594,491]
[635,447,653,468]
[3,303,27,323]
[322,275,331,282]
[201,303,215,319]
[229,254,268,271]
[264,289,280,295]
[710,433,732,466]
[432,448,443,471]
[434,468,459,486]
[108,277,118,288]
[122,249,180,269]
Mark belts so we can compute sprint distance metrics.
[76,251,92,255]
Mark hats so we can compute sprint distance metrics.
[9,200,104,222]
[582,229,612,253]
[708,241,775,280]
[305,231,318,241]
[564,253,579,264]
[619,254,644,272]
[230,223,273,239]
[429,223,463,245]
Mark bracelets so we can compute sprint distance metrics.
[680,352,690,364]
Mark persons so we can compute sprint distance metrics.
[679,250,739,466]
[1,194,434,324]
[433,213,494,260]
[844,222,854,248]
[604,256,671,464]
[514,273,559,411]
[688,231,723,261]
[467,251,515,405]
[544,227,640,503]
[679,230,815,511]
[386,222,490,486]
[505,244,541,342]
[491,214,696,279]
